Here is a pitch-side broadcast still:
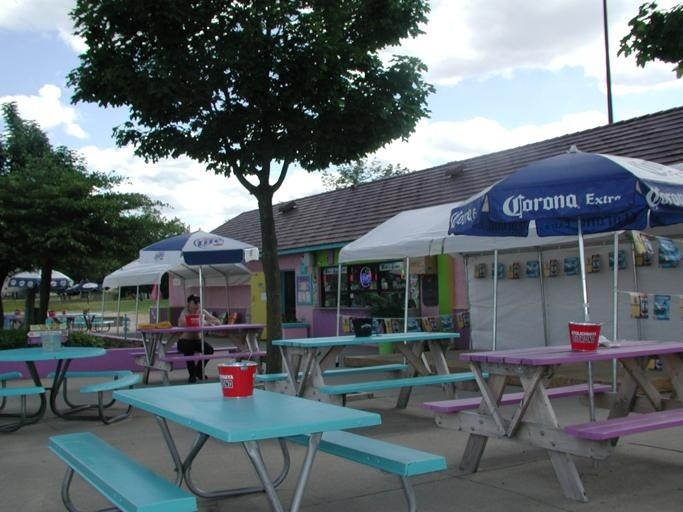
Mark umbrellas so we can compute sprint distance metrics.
[6,267,73,293]
[138,228,259,380]
[447,145,683,423]
[65,280,111,311]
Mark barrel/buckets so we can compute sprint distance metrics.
[217,362,258,397]
[568,320,602,352]
[40,331,61,351]
[379,342,395,356]
[185,314,200,326]
[351,317,372,337]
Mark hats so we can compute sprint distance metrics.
[187,294,200,301]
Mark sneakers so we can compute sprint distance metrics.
[188,373,197,384]
[197,372,207,383]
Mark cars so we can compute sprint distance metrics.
[65,281,103,297]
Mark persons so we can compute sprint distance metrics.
[176,293,221,384]
[14,308,23,325]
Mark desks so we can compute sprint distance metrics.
[57,314,97,331]
